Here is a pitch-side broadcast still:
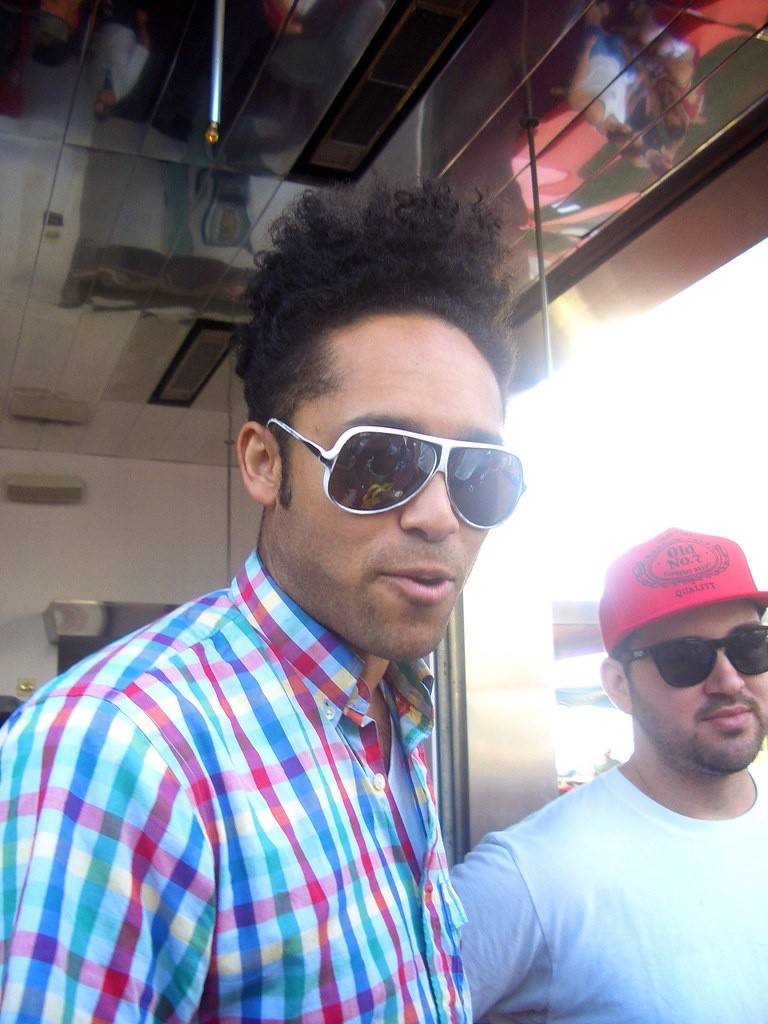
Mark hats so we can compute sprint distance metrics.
[598,527,768,655]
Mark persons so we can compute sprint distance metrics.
[87,0,150,124]
[568,0,704,176]
[0,177,524,1024]
[449,527,768,1024]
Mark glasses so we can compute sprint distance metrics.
[609,626,768,687]
[266,417,527,529]
